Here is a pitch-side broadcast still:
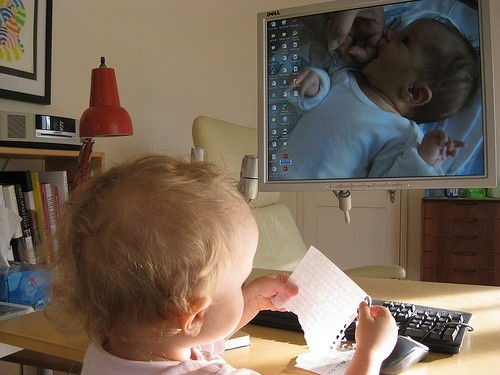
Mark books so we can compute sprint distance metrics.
[0,170,69,266]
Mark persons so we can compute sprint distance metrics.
[324,5,387,67]
[270,17,485,181]
[56,151,399,375]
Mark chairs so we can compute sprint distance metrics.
[192,116,406,281]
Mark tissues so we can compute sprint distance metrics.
[0,205,62,314]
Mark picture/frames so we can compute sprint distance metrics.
[0,0,54,104]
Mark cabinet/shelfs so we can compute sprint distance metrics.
[423,198,500,286]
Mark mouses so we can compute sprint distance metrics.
[379,335,430,375]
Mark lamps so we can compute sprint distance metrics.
[75,57,134,190]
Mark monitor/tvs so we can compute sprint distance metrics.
[256,0,498,192]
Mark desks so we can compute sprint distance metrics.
[0,269,500,374]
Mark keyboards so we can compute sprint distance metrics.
[248,297,473,353]
